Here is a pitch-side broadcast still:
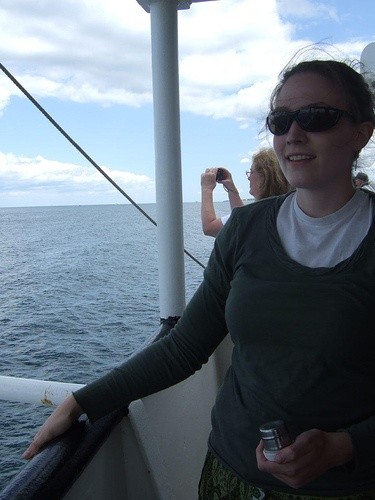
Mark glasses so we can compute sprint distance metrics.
[245,170,261,178]
[267,107,354,136]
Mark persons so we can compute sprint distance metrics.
[200,148,292,237]
[22,60,375,500]
[355,173,375,193]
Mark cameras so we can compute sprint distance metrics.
[216,173,223,181]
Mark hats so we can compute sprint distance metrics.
[352,173,368,181]
